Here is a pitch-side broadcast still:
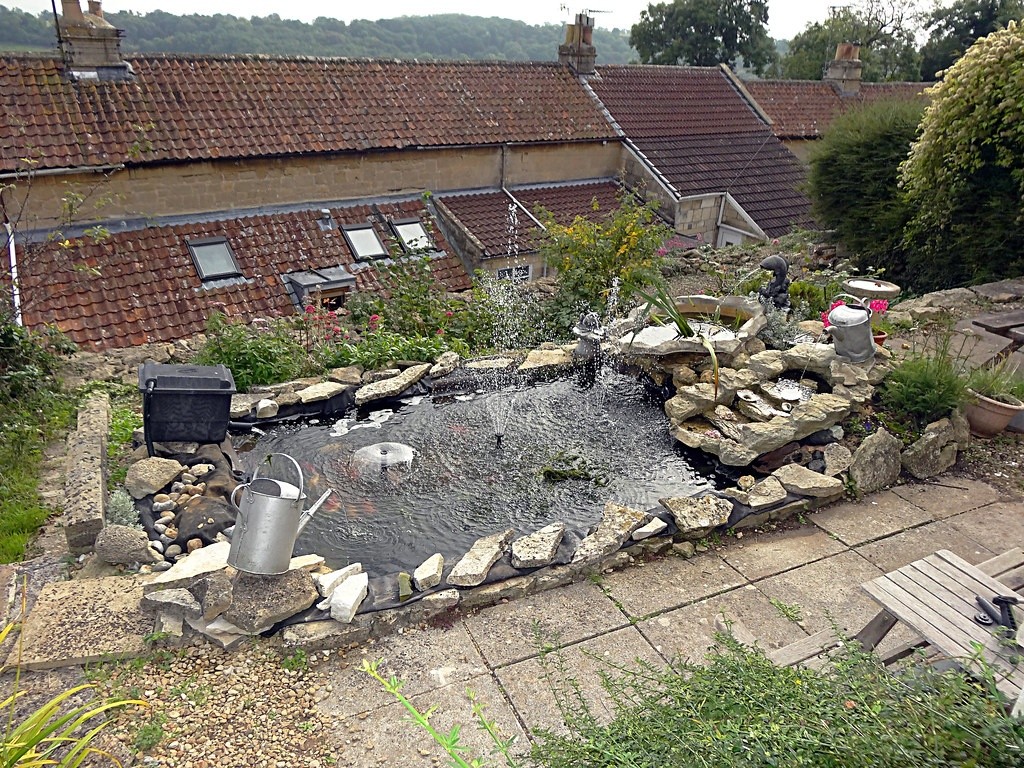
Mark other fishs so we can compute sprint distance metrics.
[303,440,378,520]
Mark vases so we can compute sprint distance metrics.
[873,330,888,346]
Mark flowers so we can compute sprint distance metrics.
[821,298,889,333]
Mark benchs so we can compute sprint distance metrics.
[973,547,1024,595]
[767,627,849,681]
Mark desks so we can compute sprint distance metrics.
[852,549,1024,706]
[971,309,1024,369]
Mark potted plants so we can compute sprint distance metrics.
[960,348,1024,438]
